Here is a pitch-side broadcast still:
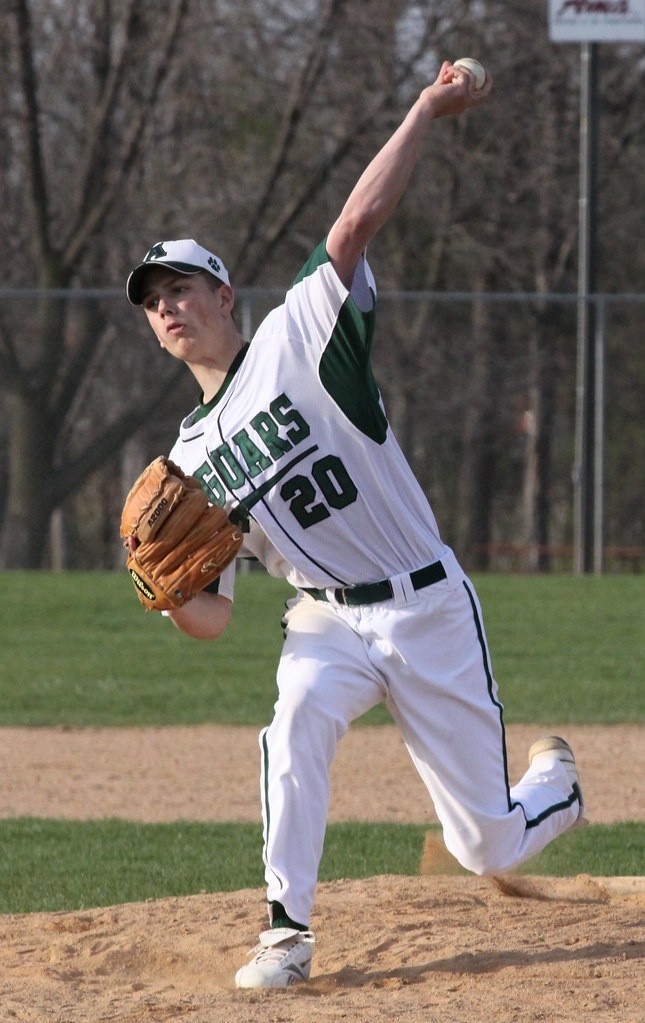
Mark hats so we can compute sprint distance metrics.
[124,237,229,308]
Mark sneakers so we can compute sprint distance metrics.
[527,737,584,832]
[235,928,316,988]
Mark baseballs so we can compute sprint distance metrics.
[453,56,487,93]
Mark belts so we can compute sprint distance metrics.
[303,563,448,606]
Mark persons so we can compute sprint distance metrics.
[119,57,586,989]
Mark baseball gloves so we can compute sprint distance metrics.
[119,455,244,612]
